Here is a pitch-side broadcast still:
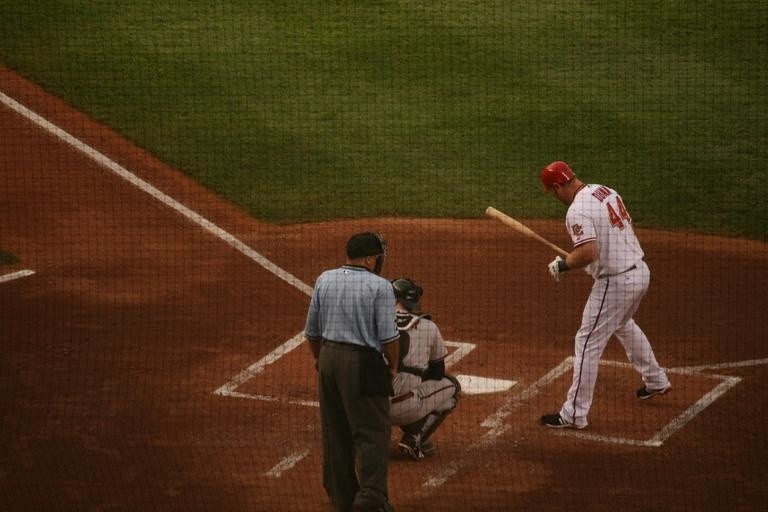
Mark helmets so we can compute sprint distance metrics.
[389,278,423,312]
[346,230,385,275]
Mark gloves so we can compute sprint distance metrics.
[547,255,565,282]
[581,262,593,276]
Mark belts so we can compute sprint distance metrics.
[321,340,370,352]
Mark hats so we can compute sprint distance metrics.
[540,161,576,192]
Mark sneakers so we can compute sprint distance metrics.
[637,381,672,400]
[400,431,435,456]
[540,412,587,430]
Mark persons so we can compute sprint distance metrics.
[385,275,461,460]
[540,160,674,429]
[306,231,403,511]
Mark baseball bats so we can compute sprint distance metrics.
[485,206,586,270]
